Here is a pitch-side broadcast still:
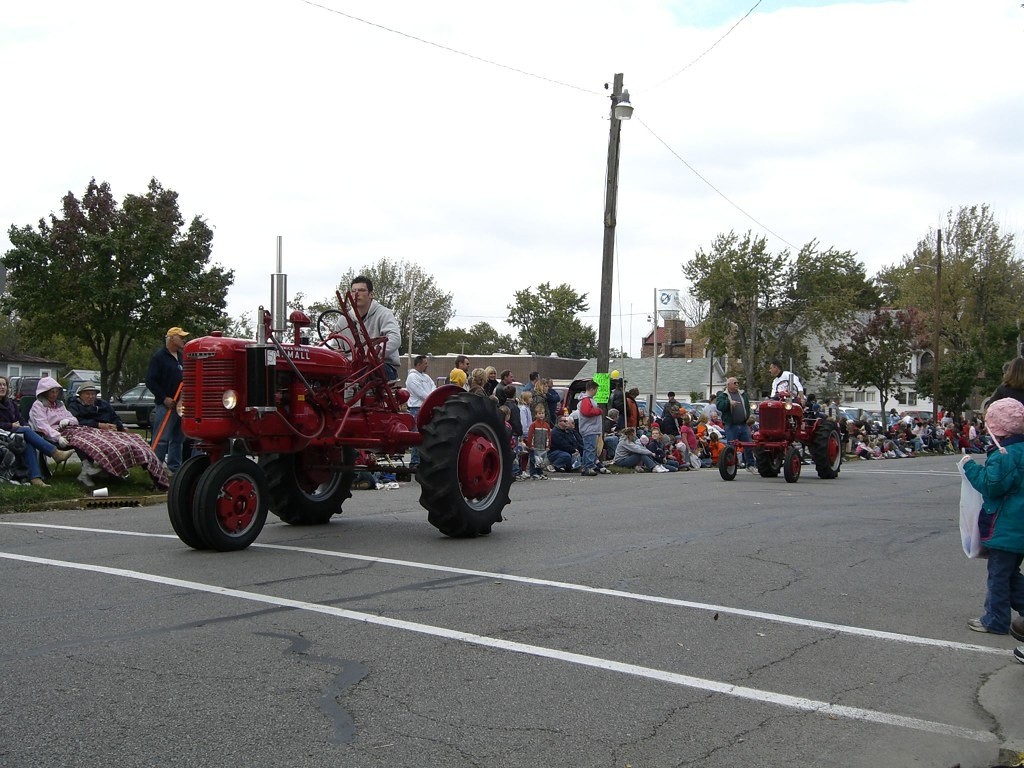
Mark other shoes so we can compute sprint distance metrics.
[582,469,598,476]
[54,449,75,464]
[546,465,556,472]
[77,461,102,487]
[652,465,669,473]
[32,479,51,487]
[1009,616,1024,642]
[531,474,548,480]
[1014,646,1024,663]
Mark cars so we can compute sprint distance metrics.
[74,385,114,403]
[108,386,158,428]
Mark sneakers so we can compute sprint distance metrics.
[599,466,611,474]
[746,466,760,475]
[968,617,990,632]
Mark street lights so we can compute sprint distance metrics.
[914,263,941,424]
[595,90,635,460]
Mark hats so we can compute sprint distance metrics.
[985,398,1024,437]
[77,382,100,395]
[167,327,190,337]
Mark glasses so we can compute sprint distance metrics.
[560,420,568,423]
[732,382,740,385]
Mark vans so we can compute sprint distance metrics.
[69,380,90,396]
[634,400,709,420]
[8,376,44,411]
[436,375,570,418]
[835,404,934,426]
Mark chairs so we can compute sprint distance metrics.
[22,394,155,480]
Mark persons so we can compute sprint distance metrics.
[67,380,170,492]
[28,377,103,487]
[405,355,993,483]
[145,327,203,474]
[960,398,1024,635]
[0,376,76,488]
[1009,616,1024,664]
[980,355,1024,409]
[320,275,402,412]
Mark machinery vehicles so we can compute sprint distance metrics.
[167,235,520,553]
[718,357,844,482]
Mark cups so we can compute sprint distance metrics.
[93,487,108,497]
